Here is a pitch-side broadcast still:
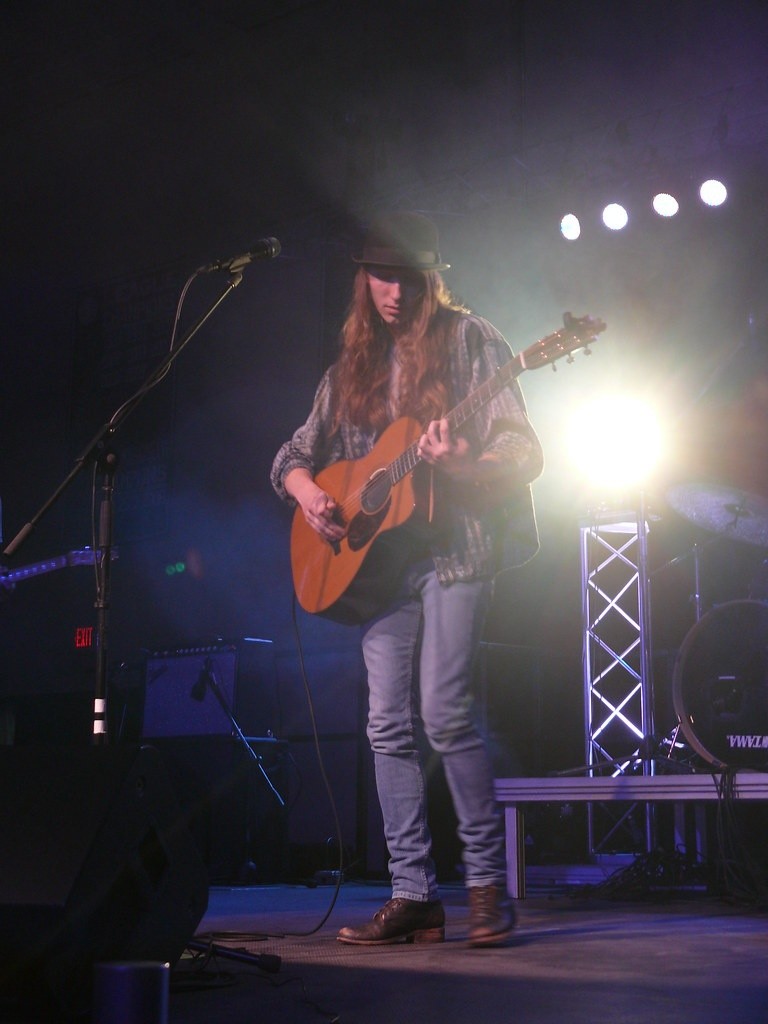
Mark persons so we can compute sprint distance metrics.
[271,209,544,945]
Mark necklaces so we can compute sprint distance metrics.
[389,349,401,368]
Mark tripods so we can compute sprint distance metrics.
[548,524,709,780]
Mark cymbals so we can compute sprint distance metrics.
[658,479,768,547]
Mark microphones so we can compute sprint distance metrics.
[197,237,281,273]
[195,658,211,700]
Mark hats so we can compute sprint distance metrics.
[351,211,451,270]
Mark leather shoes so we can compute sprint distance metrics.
[337,898,446,945]
[464,885,516,945]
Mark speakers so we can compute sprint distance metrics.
[0,544,391,984]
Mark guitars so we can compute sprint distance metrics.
[287,307,608,626]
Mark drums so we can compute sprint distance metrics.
[670,594,768,771]
